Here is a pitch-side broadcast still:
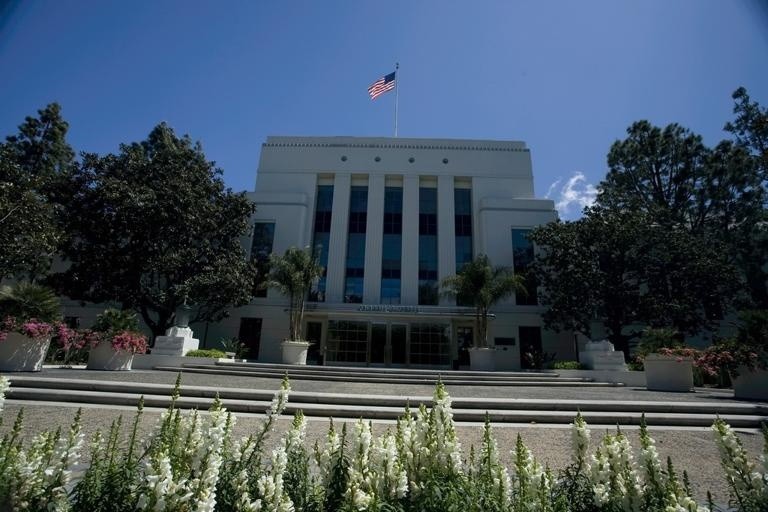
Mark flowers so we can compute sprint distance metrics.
[723,308,768,375]
[88,308,148,355]
[634,327,702,364]
[0,278,61,339]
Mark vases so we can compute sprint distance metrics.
[642,352,694,391]
[1,331,53,370]
[89,340,133,370]
[727,364,767,402]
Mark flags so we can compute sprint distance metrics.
[367,69,396,100]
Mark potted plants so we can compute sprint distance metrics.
[260,247,324,365]
[439,251,528,371]
